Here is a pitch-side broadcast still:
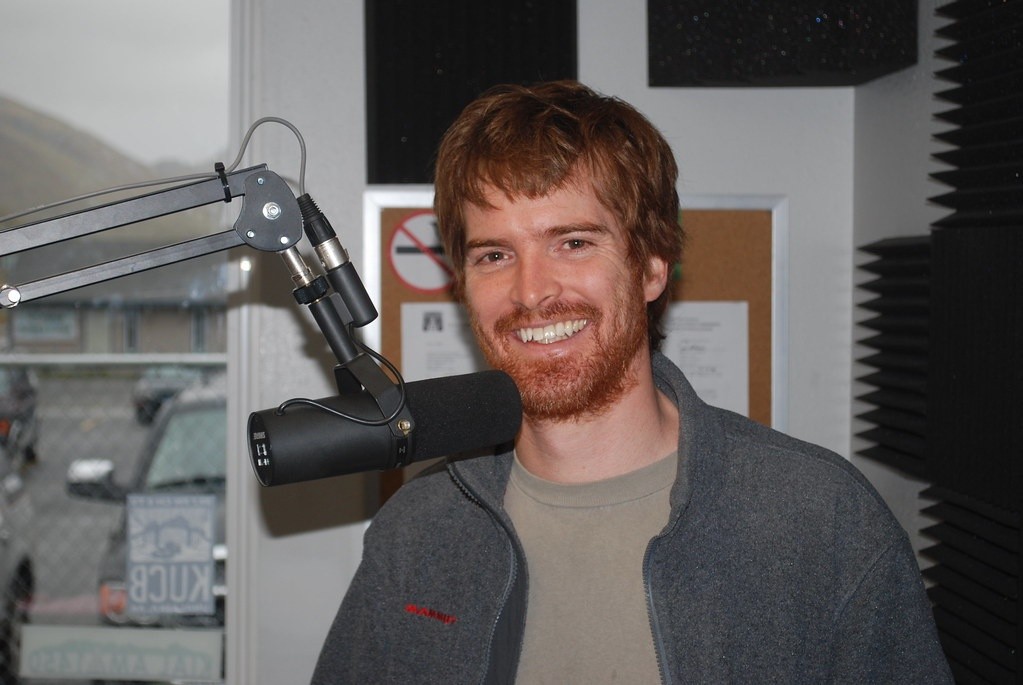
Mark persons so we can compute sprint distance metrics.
[307,81,952,685]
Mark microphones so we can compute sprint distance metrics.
[245,371,523,488]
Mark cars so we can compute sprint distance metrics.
[134,367,206,426]
[0,360,42,498]
[62,387,227,628]
[0,448,41,685]
